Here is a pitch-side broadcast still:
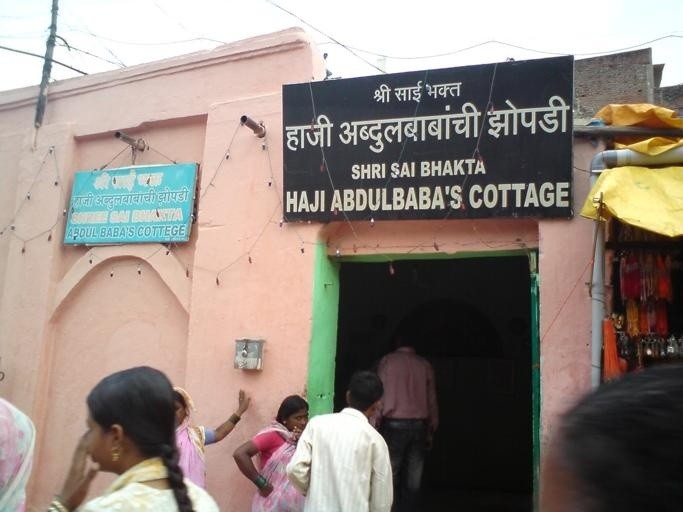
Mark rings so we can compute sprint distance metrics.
[239,398,242,399]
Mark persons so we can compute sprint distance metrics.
[231,394,309,512]
[169,383,252,490]
[538,362,682,512]
[0,376,34,512]
[43,365,222,512]
[286,371,393,511]
[366,320,439,507]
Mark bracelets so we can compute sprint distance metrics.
[227,413,240,424]
[253,474,268,489]
[47,499,67,512]
